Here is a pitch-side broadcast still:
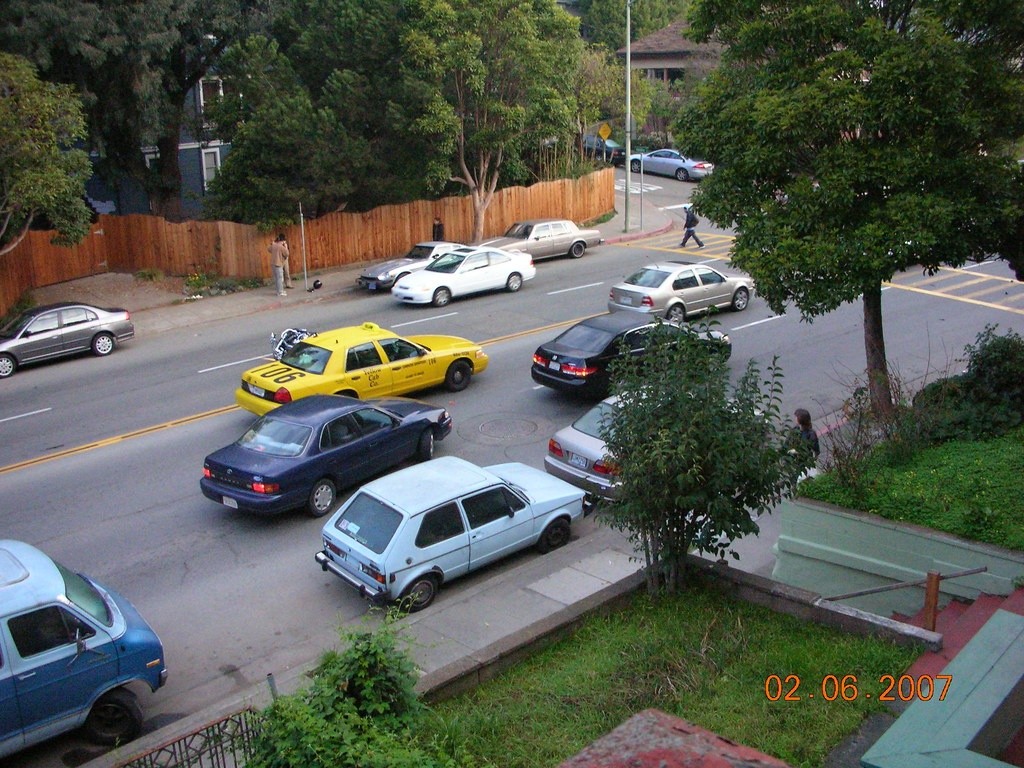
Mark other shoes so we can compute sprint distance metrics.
[286,286,294,289]
[278,291,287,296]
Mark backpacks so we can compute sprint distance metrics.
[690,213,699,227]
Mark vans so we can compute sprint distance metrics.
[0,539,168,758]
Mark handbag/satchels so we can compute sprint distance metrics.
[796,450,821,489]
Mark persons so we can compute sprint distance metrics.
[269,237,289,296]
[278,234,294,289]
[775,408,821,481]
[432,217,445,242]
[678,205,705,249]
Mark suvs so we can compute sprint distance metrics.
[314,455,585,612]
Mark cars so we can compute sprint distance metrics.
[630,149,714,181]
[199,394,452,518]
[235,321,488,416]
[355,241,465,290]
[466,218,601,262]
[545,385,768,503]
[607,261,754,323]
[391,246,536,308]
[582,135,626,166]
[0,302,134,378]
[530,312,732,403]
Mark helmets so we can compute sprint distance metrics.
[314,280,322,289]
